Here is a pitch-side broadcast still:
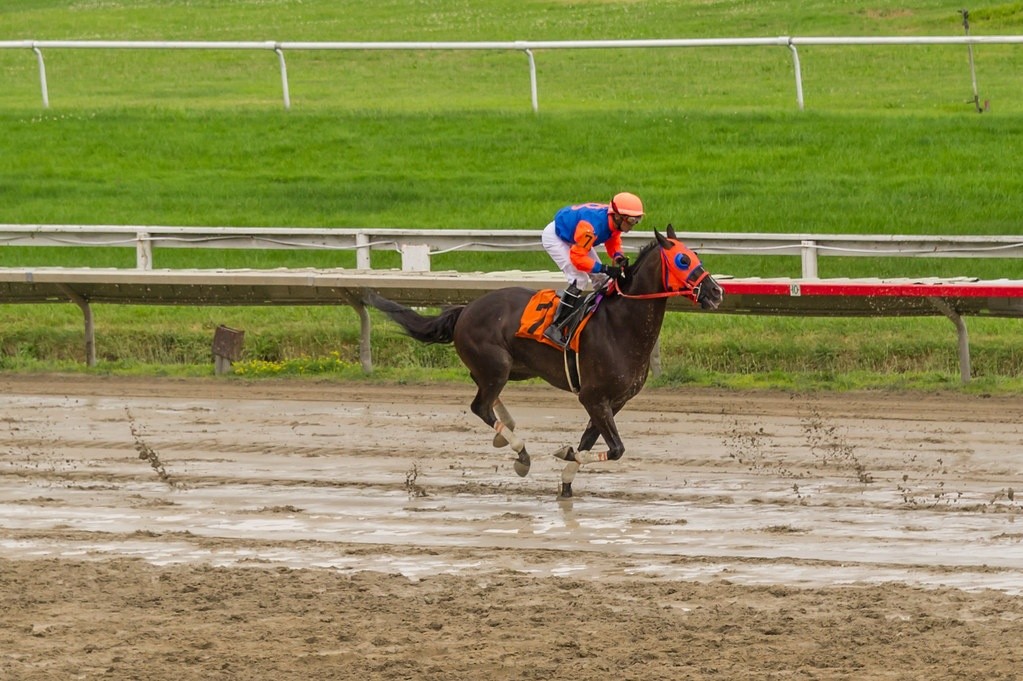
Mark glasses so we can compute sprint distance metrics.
[622,216,642,225]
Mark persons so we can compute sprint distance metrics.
[542,192,646,350]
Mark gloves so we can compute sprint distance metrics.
[616,255,630,269]
[600,264,627,283]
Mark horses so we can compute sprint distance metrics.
[368,223,724,502]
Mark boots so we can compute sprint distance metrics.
[543,289,582,351]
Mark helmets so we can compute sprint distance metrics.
[608,192,646,218]
